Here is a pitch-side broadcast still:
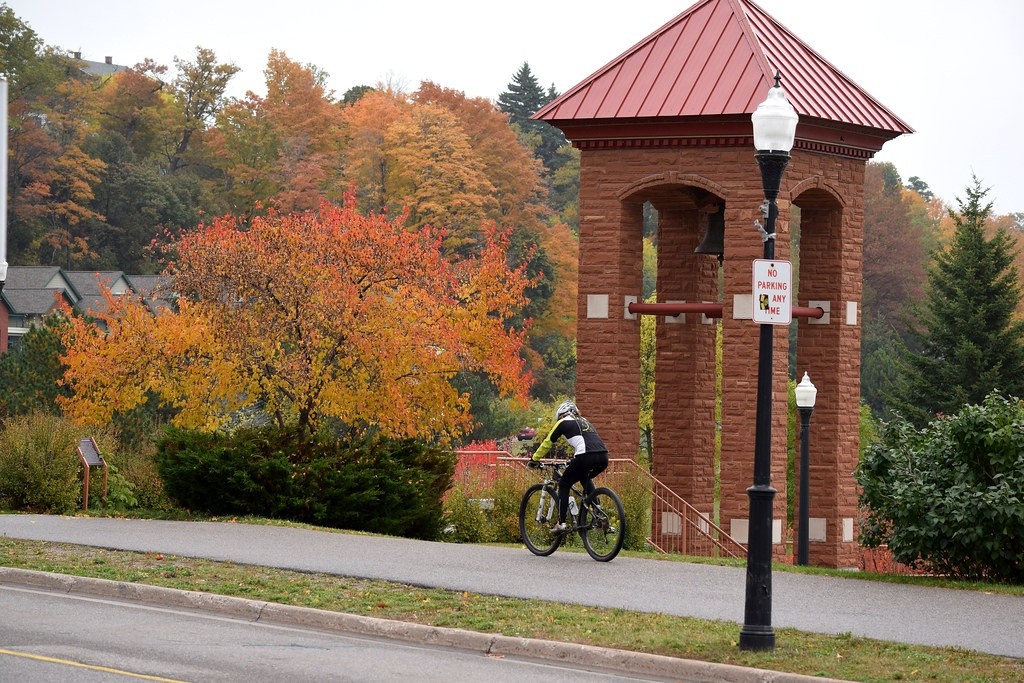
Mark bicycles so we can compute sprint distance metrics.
[519,455,626,562]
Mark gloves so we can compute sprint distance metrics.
[527,460,540,469]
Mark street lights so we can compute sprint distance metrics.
[740,70,800,652]
[794,372,817,567]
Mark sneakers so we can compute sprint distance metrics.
[549,521,567,532]
[592,505,603,518]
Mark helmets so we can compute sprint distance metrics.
[556,401,579,421]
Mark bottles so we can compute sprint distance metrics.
[569,494,579,516]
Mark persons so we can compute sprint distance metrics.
[526,400,609,534]
